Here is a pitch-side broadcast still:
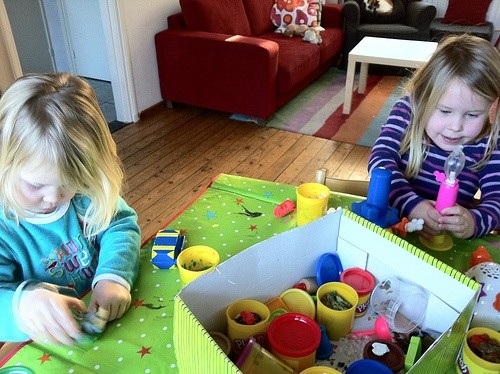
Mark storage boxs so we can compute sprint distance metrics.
[173,209,484,374]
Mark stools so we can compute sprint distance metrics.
[430,18,493,42]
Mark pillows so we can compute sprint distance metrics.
[270,0,322,33]
[441,0,493,26]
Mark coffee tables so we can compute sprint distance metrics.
[343,36,438,114]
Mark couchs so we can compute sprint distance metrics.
[154,0,343,127]
[342,0,437,77]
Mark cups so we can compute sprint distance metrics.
[340,267,372,318]
[299,365,342,374]
[176,246,220,289]
[370,275,430,334]
[225,298,270,354]
[296,182,331,227]
[234,336,294,374]
[266,312,321,372]
[345,340,406,374]
[317,281,358,342]
[455,327,500,374]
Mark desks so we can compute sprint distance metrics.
[0,173,500,374]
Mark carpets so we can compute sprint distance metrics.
[228,67,405,147]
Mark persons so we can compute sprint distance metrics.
[367,34,500,240]
[0,72,141,346]
